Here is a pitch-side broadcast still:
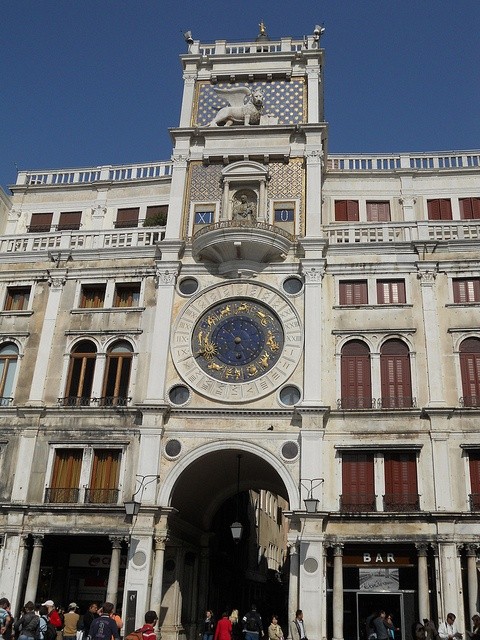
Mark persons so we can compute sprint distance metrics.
[0,598,10,640]
[135,610,158,640]
[290,610,308,640]
[242,606,264,640]
[63,602,80,640]
[374,610,399,640]
[2,601,14,639]
[268,615,285,640]
[228,609,239,640]
[384,614,396,640]
[40,606,57,640]
[438,613,463,640]
[98,606,123,640]
[83,601,101,640]
[214,612,233,640]
[198,609,216,640]
[41,599,62,640]
[86,602,121,640]
[411,619,441,640]
[465,615,480,640]
[13,601,40,640]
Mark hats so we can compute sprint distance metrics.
[251,605,256,609]
[41,600,54,607]
[68,602,79,611]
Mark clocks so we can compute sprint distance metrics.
[170,279,304,402]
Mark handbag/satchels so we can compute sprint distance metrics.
[14,631,20,639]
[300,637,308,640]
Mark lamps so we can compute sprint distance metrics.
[230,455,243,538]
[300,479,324,513]
[123,475,160,515]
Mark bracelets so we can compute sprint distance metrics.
[434,633,439,637]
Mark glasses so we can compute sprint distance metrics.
[417,626,425,631]
[451,619,454,622]
[156,617,158,620]
[388,617,391,619]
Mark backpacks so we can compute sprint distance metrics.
[40,617,57,639]
[125,627,153,640]
[246,611,259,631]
